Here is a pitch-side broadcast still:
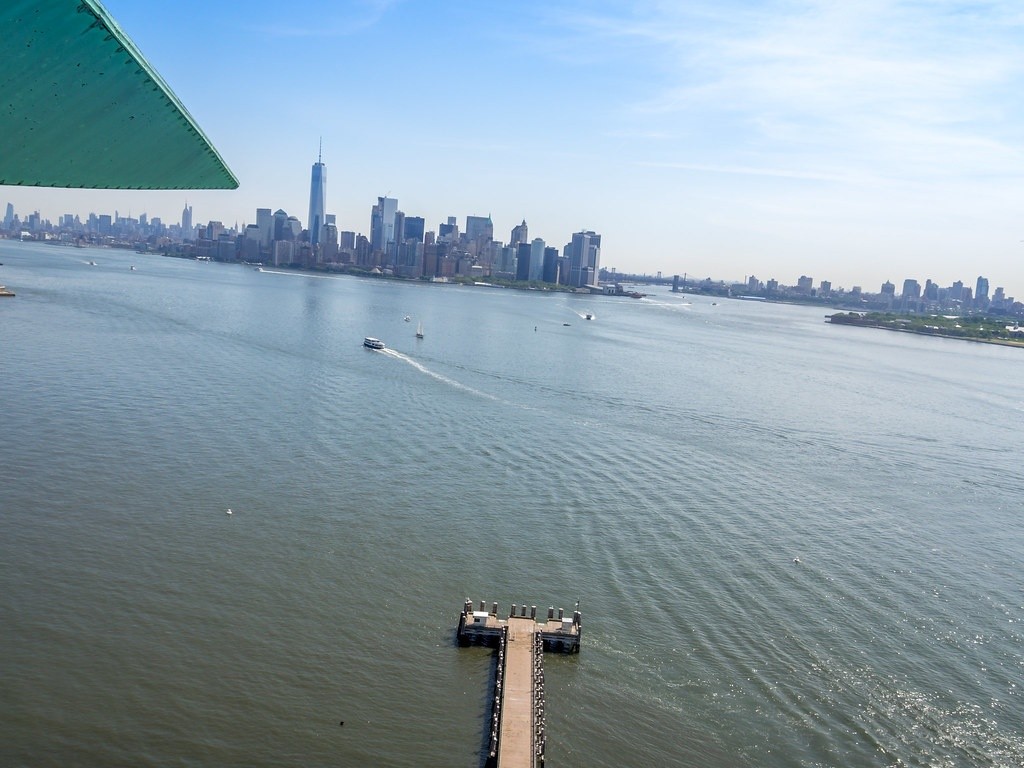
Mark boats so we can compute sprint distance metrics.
[416,321,423,338]
[586,314,592,320]
[364,337,385,349]
[404,316,410,322]
